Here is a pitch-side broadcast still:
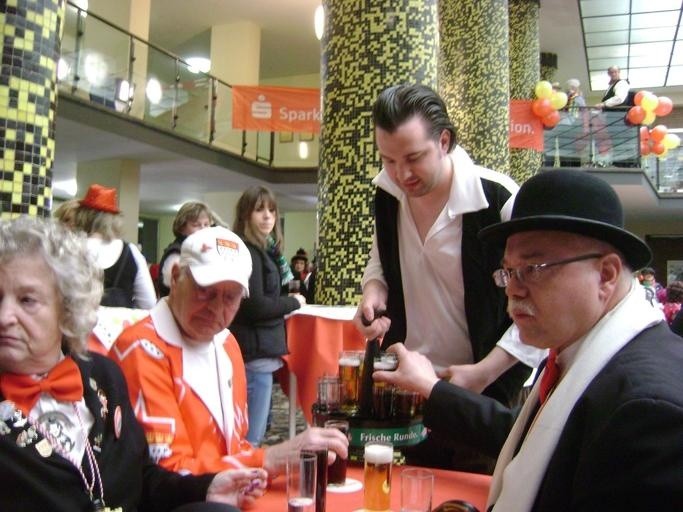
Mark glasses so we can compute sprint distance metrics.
[492,253,602,288]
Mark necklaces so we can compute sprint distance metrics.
[21,400,123,512]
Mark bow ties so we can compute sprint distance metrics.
[0,355,83,415]
[537,349,558,403]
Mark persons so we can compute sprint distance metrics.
[639,266,683,339]
[159,200,224,295]
[280,241,317,307]
[53,184,157,310]
[354,84,551,477]
[220,184,307,451]
[0,213,268,511]
[107,225,350,484]
[372,166,683,511]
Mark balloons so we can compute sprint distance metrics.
[532,65,681,162]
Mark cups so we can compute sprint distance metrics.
[400,467,434,511]
[363,440,394,512]
[285,452,318,512]
[299,441,329,512]
[324,420,349,487]
[316,350,434,428]
[288,280,301,298]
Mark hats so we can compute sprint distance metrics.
[478,169,653,272]
[179,226,252,298]
[551,79,562,90]
[78,185,120,214]
[291,248,309,261]
